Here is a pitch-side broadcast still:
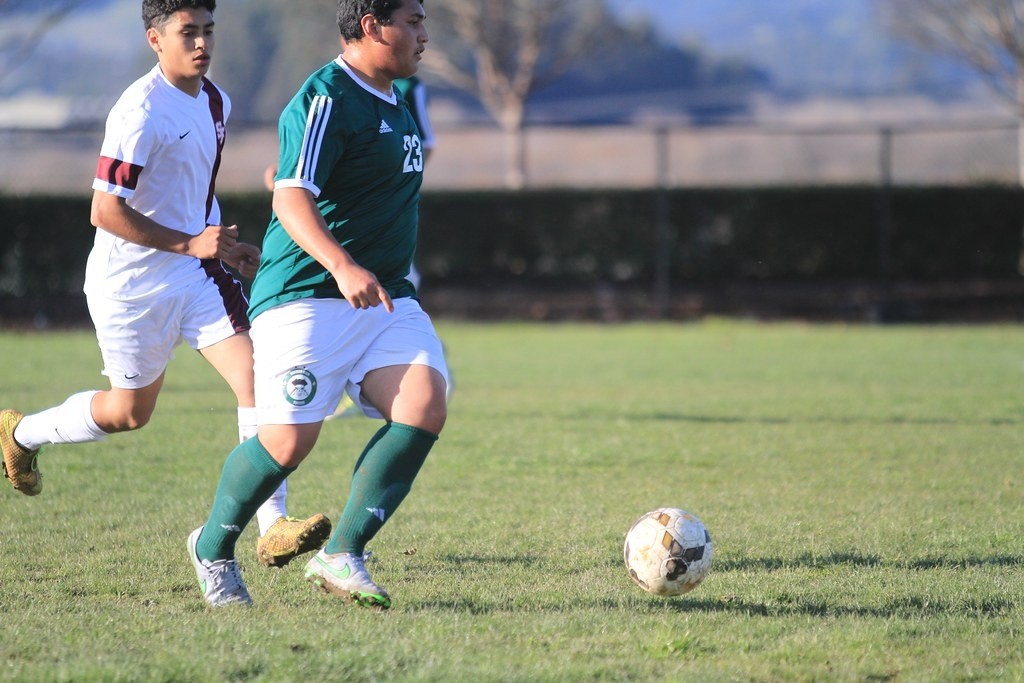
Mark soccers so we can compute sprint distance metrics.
[623,506,714,598]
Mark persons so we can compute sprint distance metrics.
[390,76,434,296]
[0,0,333,567]
[187,0,451,611]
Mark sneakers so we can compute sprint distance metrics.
[303,546,391,610]
[186,525,253,605]
[0,408,42,496]
[260,514,332,567]
